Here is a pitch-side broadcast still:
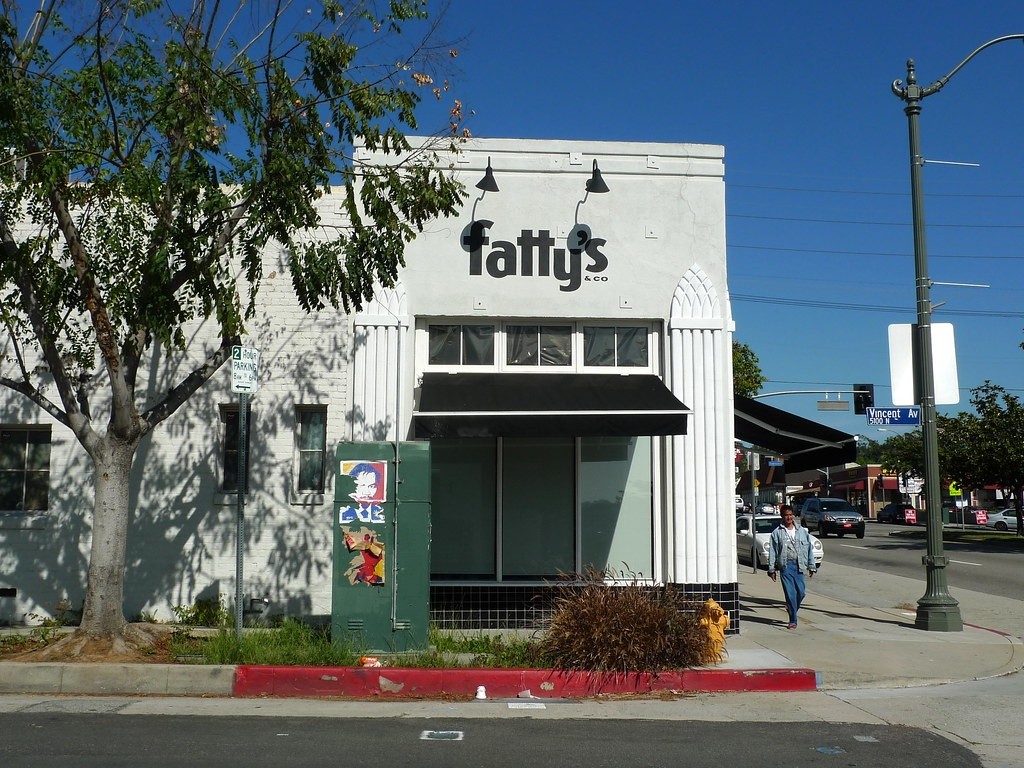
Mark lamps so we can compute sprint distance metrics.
[585,158,611,194]
[474,155,500,192]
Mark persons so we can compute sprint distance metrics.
[767,505,817,630]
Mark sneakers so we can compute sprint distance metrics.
[788,623,797,630]
[785,603,789,612]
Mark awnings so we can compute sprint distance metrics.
[785,486,820,496]
[833,480,864,490]
[413,374,694,437]
[733,393,857,475]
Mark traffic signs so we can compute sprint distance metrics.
[231,345,259,395]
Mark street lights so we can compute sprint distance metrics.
[877,429,910,506]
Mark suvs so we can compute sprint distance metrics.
[799,497,865,538]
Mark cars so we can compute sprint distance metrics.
[876,502,917,523]
[962,506,984,514]
[985,508,1024,530]
[943,502,960,513]
[735,494,775,514]
[735,514,824,571]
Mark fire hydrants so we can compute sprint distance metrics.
[695,597,732,660]
